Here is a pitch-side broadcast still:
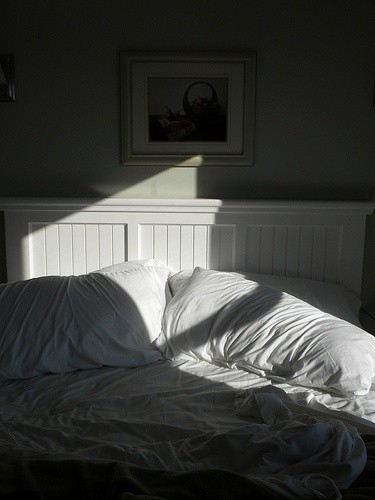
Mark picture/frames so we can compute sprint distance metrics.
[120,50,255,165]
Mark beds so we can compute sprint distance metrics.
[0,194,375,500]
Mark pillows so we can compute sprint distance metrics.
[166,270,363,330]
[151,266,374,393]
[0,257,172,381]
